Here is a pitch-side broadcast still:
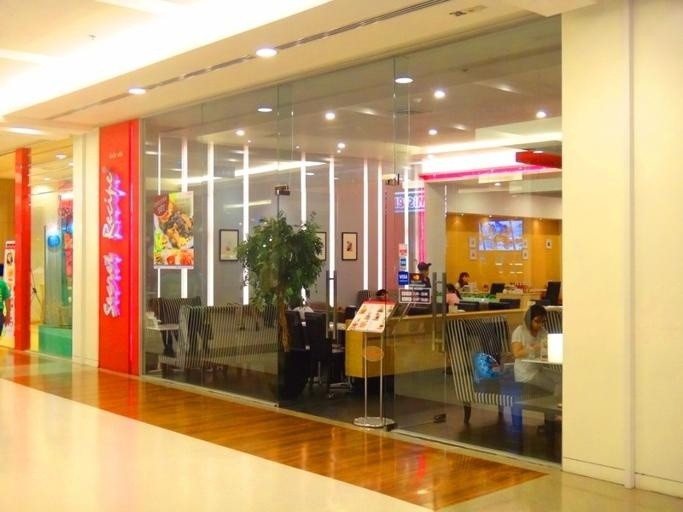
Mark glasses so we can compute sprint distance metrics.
[533,318,546,323]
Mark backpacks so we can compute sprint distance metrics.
[473,352,501,379]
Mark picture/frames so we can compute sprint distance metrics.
[220,229,358,261]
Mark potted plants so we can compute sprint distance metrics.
[234,209,325,401]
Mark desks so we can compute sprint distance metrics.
[513,396,561,454]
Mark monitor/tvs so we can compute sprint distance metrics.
[490,282,505,295]
[478,219,525,252]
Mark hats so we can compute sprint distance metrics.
[417,262,431,271]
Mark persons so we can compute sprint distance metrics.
[445,283,463,306]
[510,304,549,357]
[7,252,14,308]
[417,262,432,288]
[376,289,389,301]
[0,263,12,337]
[455,272,470,290]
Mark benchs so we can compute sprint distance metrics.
[148,296,277,381]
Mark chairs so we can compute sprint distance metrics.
[286,310,345,393]
[529,281,561,307]
[448,309,563,426]
